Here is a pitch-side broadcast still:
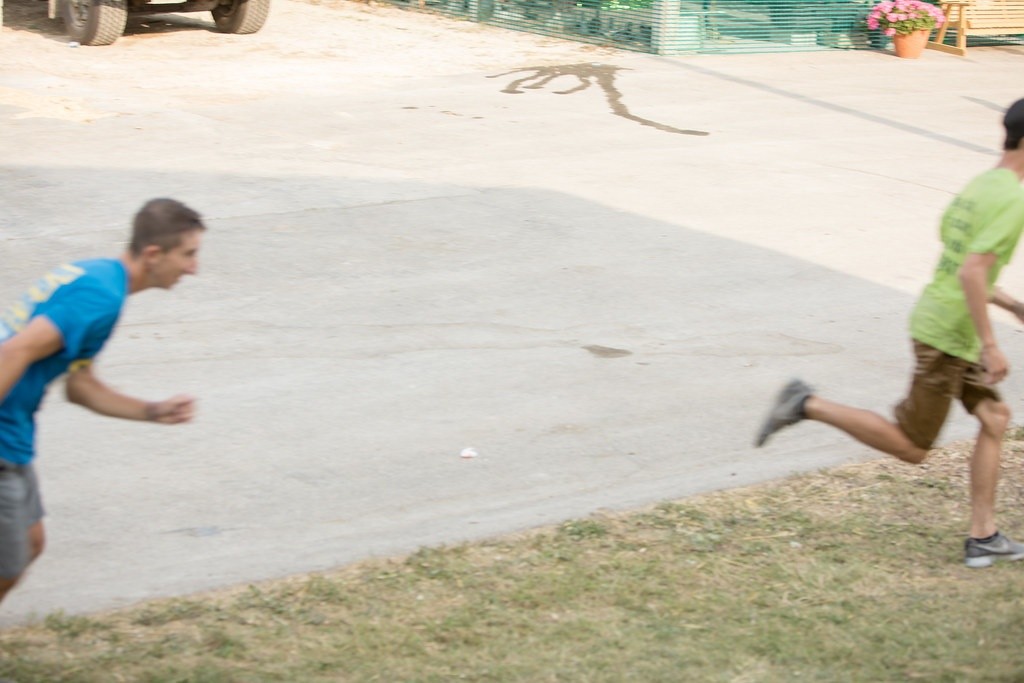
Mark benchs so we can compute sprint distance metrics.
[927,0,1024,57]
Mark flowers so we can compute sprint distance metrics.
[865,0,947,37]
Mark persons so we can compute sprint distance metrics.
[751,98,1024,569]
[0,197,209,605]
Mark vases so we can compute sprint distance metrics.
[894,30,930,59]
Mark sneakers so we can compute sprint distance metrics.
[754,380,813,447]
[965,534,1024,568]
